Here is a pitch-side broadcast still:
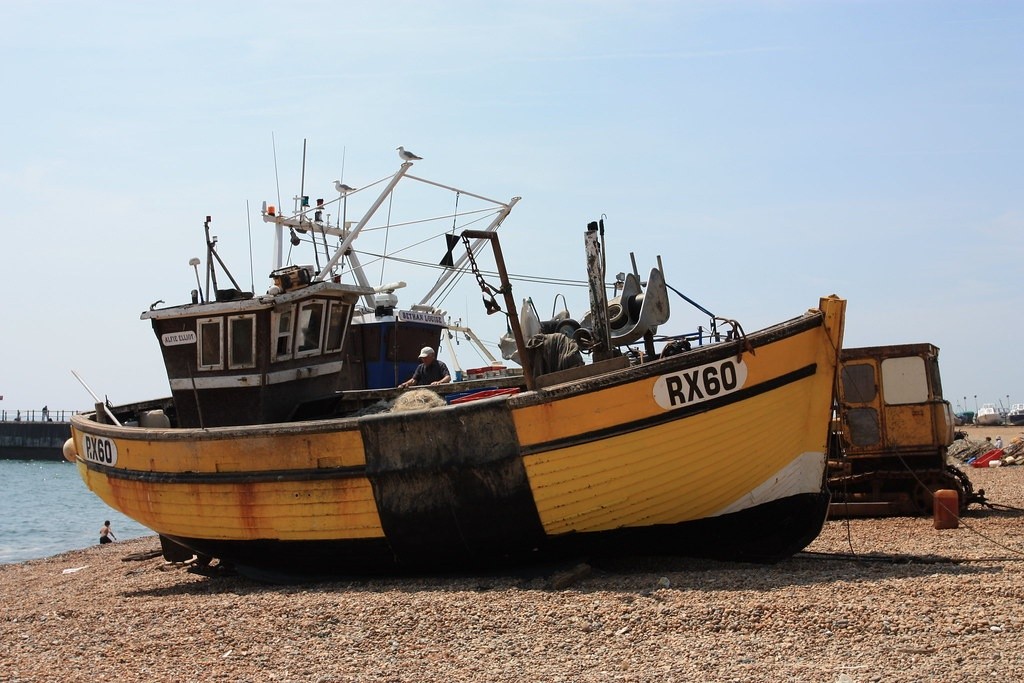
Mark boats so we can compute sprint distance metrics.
[955,411,976,423]
[1007,404,1024,426]
[62,136,845,576]
[973,404,1002,426]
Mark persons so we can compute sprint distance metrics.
[398,347,451,387]
[986,437,991,442]
[41,406,48,420]
[99,520,116,544]
[994,435,1002,448]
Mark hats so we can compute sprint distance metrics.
[996,436,1001,439]
[418,348,433,358]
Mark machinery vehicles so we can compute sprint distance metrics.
[825,344,987,513]
